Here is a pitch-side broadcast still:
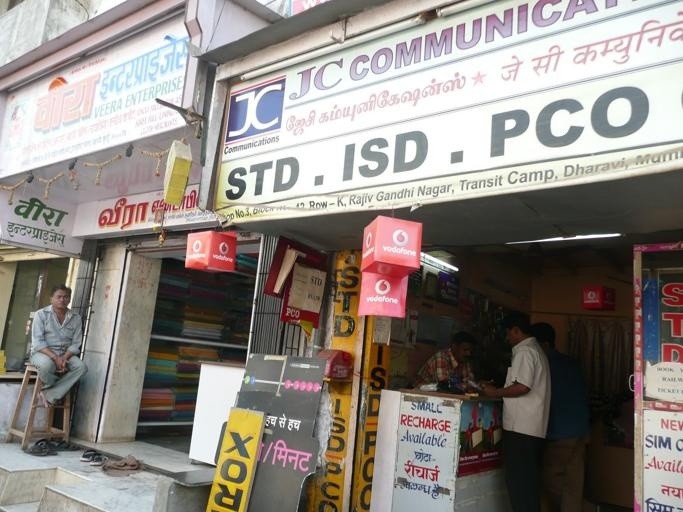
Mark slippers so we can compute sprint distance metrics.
[80,449,109,466]
[25,438,58,456]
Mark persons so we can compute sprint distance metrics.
[30,285,89,408]
[481,313,585,512]
[412,332,481,391]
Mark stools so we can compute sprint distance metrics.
[6,362,71,450]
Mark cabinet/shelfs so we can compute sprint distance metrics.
[136,258,256,427]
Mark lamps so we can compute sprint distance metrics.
[420,253,460,273]
